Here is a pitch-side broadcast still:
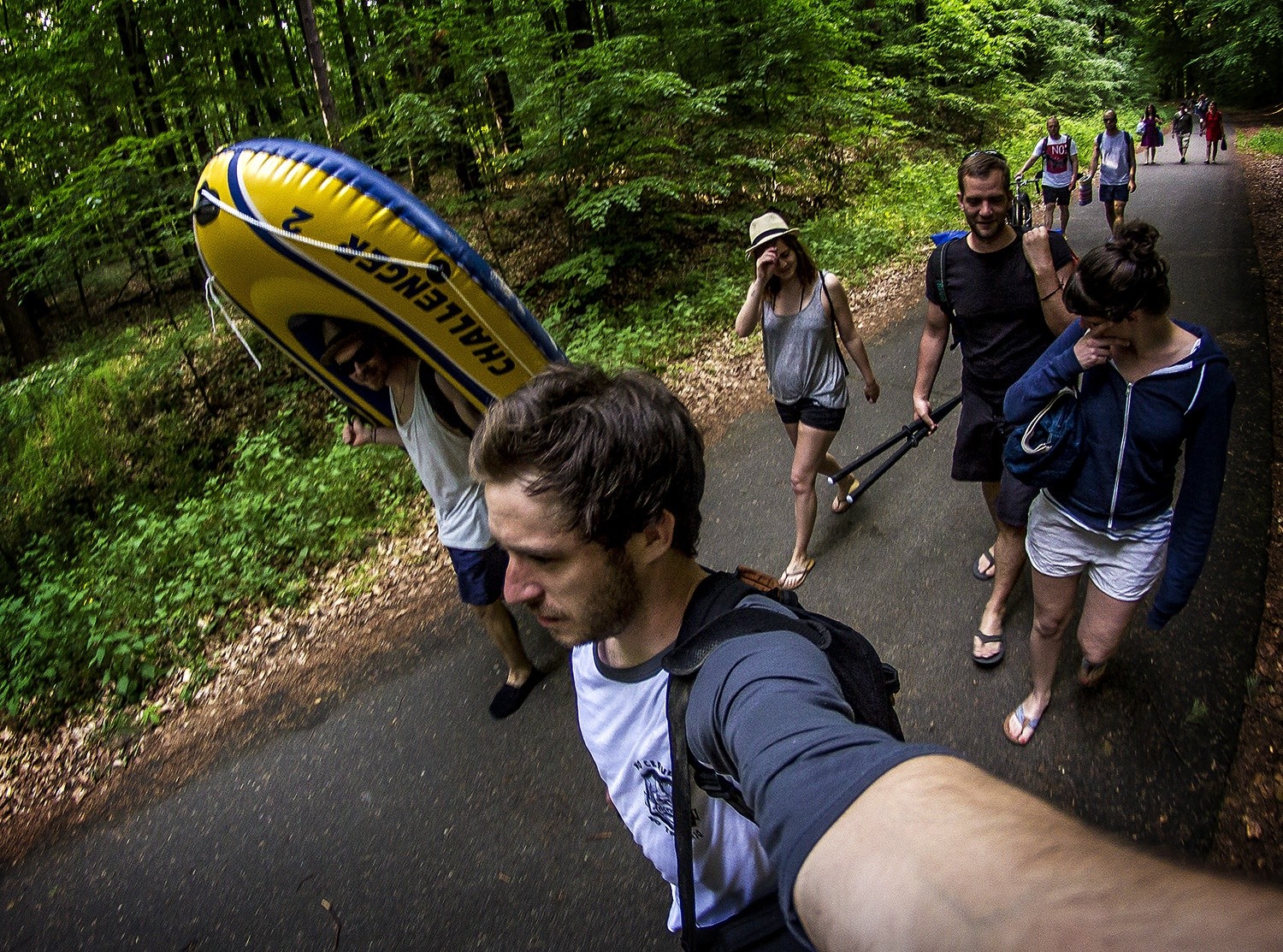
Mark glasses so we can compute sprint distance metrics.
[960,149,1008,164]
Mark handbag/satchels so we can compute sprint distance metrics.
[1158,131,1164,147]
[1220,134,1227,150]
[734,566,908,743]
[1136,118,1147,136]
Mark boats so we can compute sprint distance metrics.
[189,135,576,417]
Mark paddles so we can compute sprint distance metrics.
[827,392,964,505]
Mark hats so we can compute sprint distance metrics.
[744,212,801,253]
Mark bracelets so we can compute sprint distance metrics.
[866,381,876,387]
[1040,283,1064,301]
[371,427,377,445]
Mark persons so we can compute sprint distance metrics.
[1171,101,1192,163]
[1185,94,1209,134]
[1002,217,1232,746]
[1014,118,1079,240]
[1200,101,1225,164]
[914,150,1074,665]
[1088,109,1137,235]
[1141,104,1163,164]
[735,212,880,591]
[322,329,542,719]
[466,363,1283,952]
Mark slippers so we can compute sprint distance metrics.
[1003,694,1051,745]
[1077,656,1108,689]
[778,555,816,590]
[972,541,998,581]
[972,627,1006,665]
[831,479,860,514]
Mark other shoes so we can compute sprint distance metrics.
[489,665,542,720]
[1062,233,1067,242]
[1203,160,1217,165]
[1143,161,1156,166]
[1178,156,1186,164]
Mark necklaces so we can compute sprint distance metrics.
[395,368,407,414]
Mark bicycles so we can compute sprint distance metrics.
[1010,176,1040,228]
